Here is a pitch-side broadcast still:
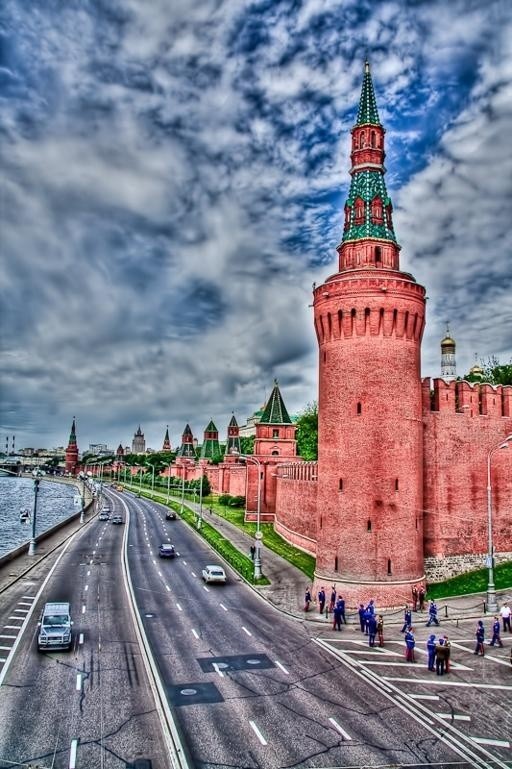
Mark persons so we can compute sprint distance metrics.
[318,587,325,614]
[328,586,336,613]
[411,585,418,612]
[498,602,511,632]
[418,586,425,610]
[303,587,311,612]
[473,620,485,656]
[332,596,451,676]
[487,616,503,648]
[250,543,256,560]
